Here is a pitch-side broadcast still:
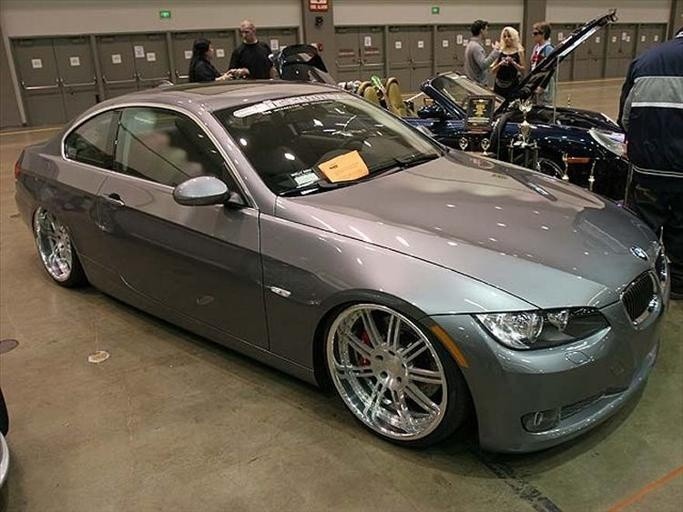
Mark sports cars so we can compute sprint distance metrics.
[267,9,627,202]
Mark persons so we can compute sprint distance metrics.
[187,39,233,83]
[529,21,556,107]
[227,21,274,80]
[617,26,682,303]
[464,19,501,89]
[490,26,527,96]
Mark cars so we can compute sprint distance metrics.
[10,81,671,454]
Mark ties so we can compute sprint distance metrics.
[532,49,538,64]
